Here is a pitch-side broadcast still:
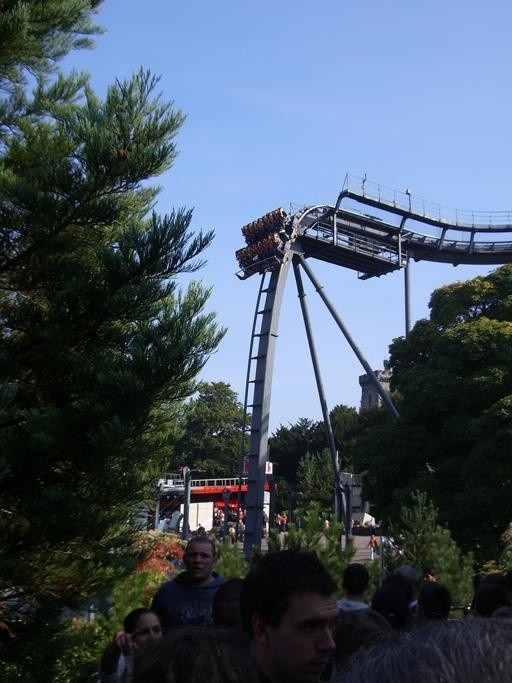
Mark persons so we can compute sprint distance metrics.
[166,507,289,545]
[235,208,288,268]
[99,535,511,682]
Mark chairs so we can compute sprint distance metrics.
[235,207,291,272]
